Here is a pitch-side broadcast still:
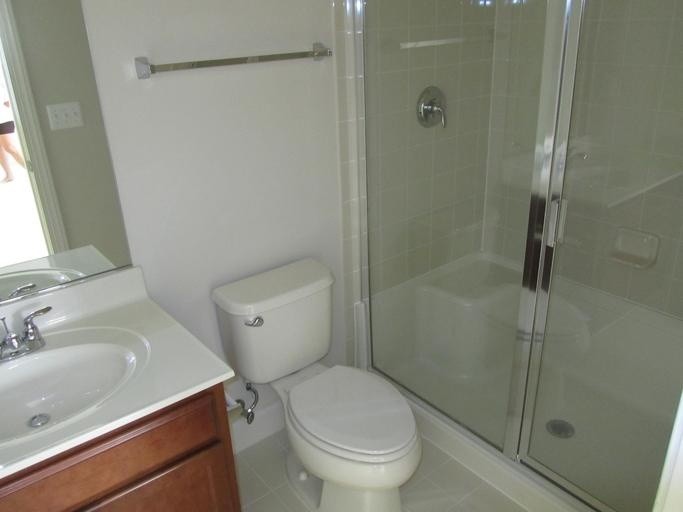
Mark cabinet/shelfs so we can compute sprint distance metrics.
[0,383,239,512]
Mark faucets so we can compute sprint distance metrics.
[2,332,22,350]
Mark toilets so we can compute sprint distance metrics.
[211,258,422,511]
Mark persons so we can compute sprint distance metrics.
[0,84,25,187]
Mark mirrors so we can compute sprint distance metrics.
[0,0,135,302]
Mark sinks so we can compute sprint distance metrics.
[0,269,87,302]
[0,325,150,446]
